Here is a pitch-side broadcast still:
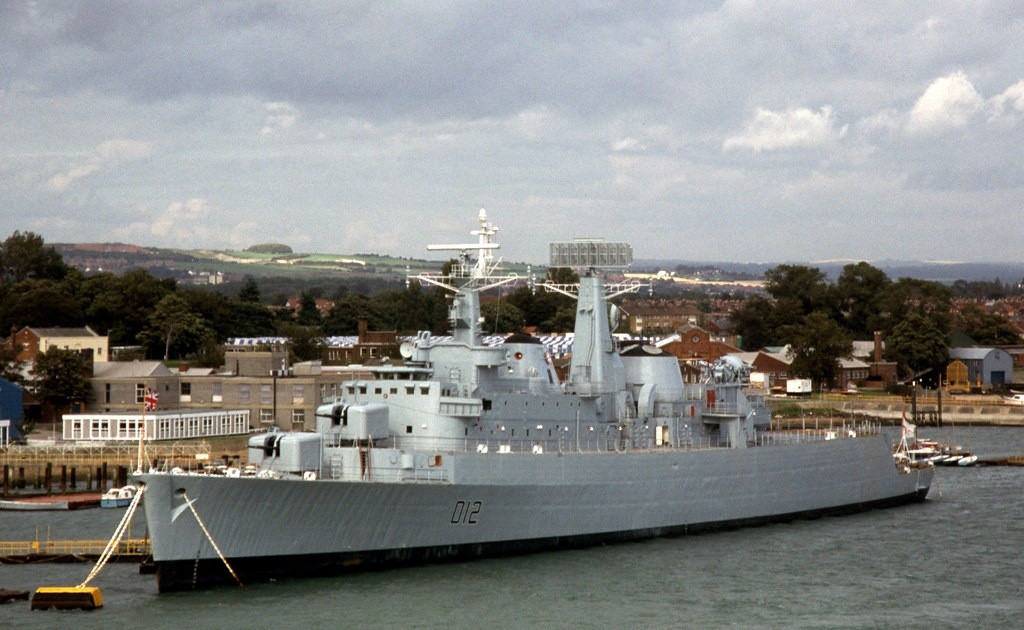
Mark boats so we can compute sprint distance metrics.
[126,274,935,595]
[893,439,978,467]
[100,484,136,509]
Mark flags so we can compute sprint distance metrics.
[144,385,159,412]
